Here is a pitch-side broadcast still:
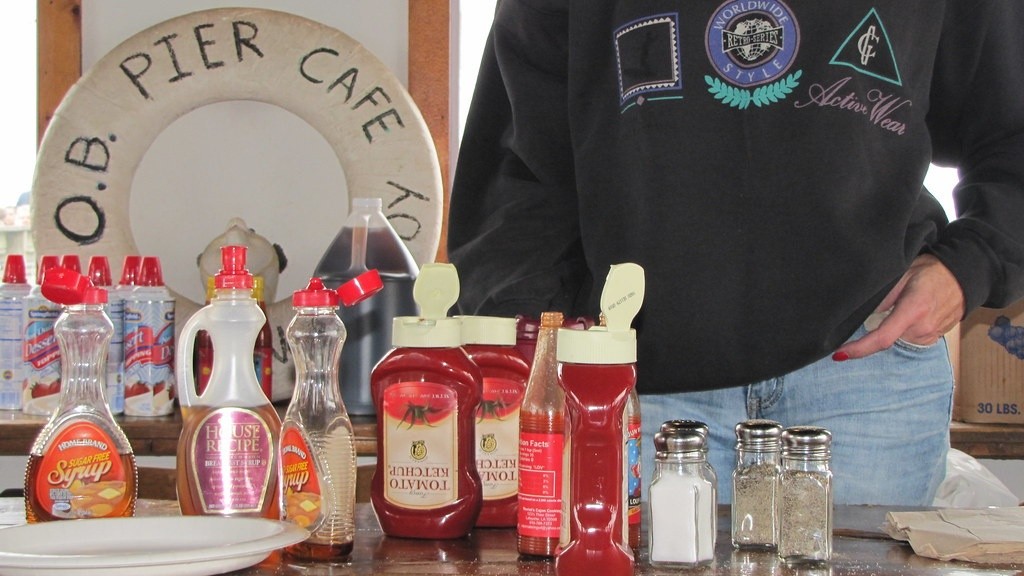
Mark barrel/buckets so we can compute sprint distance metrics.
[312,197,423,415]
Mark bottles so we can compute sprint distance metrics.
[646,420,832,569]
[1,253,645,576]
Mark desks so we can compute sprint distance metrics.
[1,393,393,499]
[2,500,1022,576]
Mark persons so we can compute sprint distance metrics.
[443,1,1024,511]
